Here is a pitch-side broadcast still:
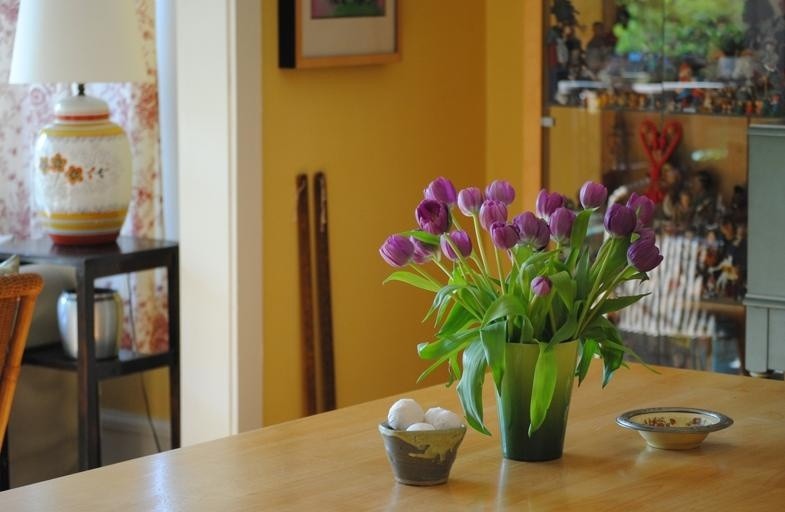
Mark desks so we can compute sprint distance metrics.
[0,352,785,512]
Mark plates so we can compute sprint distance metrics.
[617,407,735,451]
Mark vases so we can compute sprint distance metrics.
[495,338,580,461]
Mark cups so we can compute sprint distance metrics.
[380,419,466,486]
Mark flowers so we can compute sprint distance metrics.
[376,179,662,437]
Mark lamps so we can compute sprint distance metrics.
[8,0,148,244]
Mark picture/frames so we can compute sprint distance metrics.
[279,0,405,69]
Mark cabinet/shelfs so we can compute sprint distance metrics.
[0,234,180,486]
